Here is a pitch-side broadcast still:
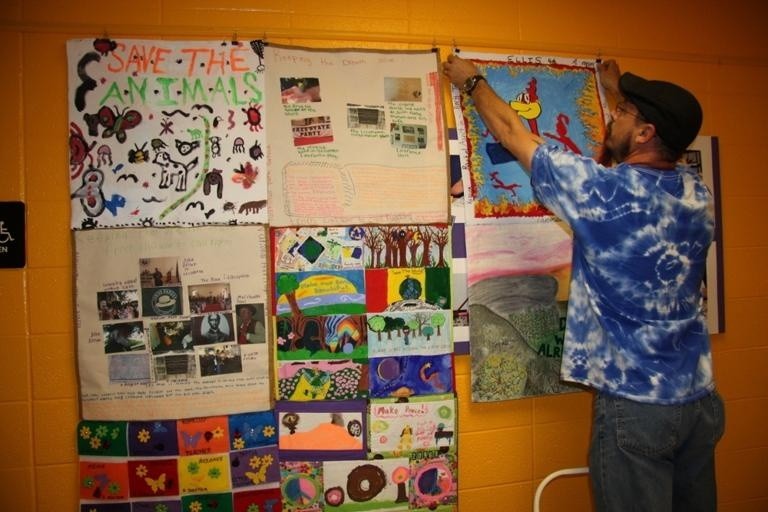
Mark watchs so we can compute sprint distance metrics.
[461,73,487,97]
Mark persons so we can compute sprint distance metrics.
[236,304,265,344]
[99,267,241,377]
[439,54,727,510]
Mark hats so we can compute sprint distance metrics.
[619,71,703,149]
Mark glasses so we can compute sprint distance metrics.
[616,101,646,124]
